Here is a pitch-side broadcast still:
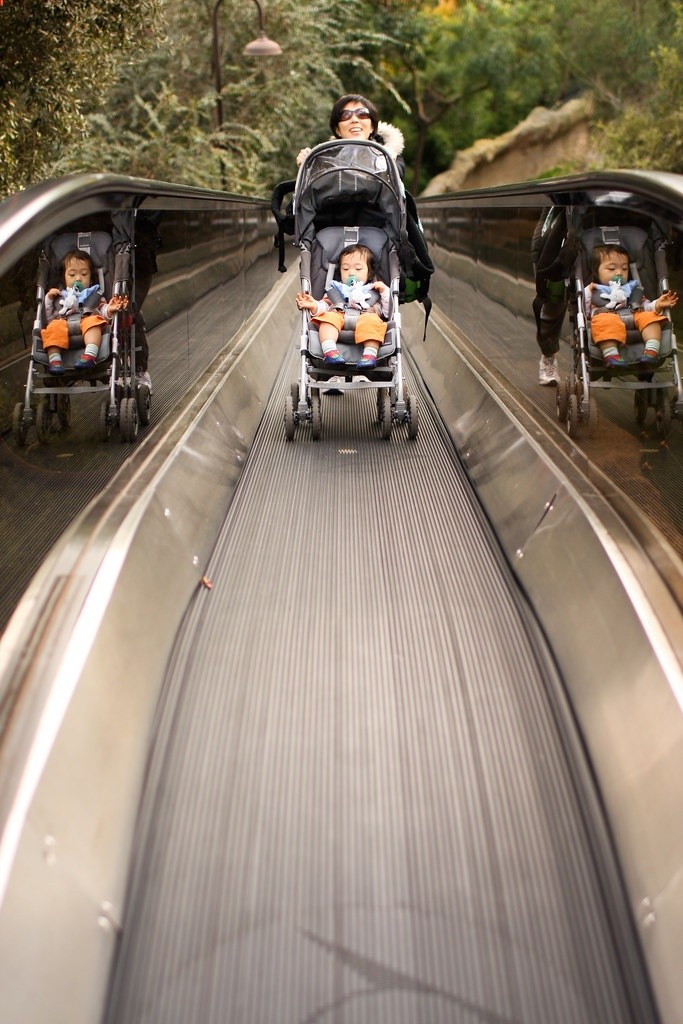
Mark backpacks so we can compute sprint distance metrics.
[398,189,435,305]
[542,208,570,308]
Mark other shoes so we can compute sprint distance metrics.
[641,354,657,363]
[357,354,375,368]
[48,360,65,375]
[323,353,346,365]
[606,355,626,368]
[74,357,95,369]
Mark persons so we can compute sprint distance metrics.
[294,93,405,396]
[37,249,129,375]
[295,243,391,369]
[578,245,678,374]
[529,205,573,386]
[130,210,163,394]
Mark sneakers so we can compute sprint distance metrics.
[354,377,368,383]
[138,371,153,394]
[538,355,560,386]
[321,377,343,395]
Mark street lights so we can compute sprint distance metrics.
[213,0,283,192]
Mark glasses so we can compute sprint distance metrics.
[338,108,370,122]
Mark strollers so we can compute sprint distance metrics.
[12,206,153,448]
[556,202,683,438]
[270,137,436,444]
